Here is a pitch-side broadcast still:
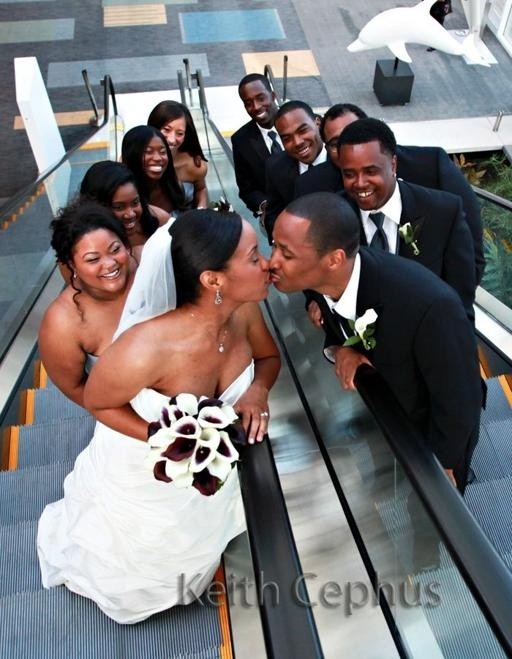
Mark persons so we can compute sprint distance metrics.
[421,0,452,52]
[36,74,487,624]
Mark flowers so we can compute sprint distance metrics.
[397,222,420,257]
[147,392,247,497]
[208,195,235,215]
[342,308,379,350]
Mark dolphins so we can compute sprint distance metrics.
[344,1,493,69]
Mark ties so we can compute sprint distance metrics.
[369,212,390,252]
[267,131,282,154]
[332,309,355,338]
